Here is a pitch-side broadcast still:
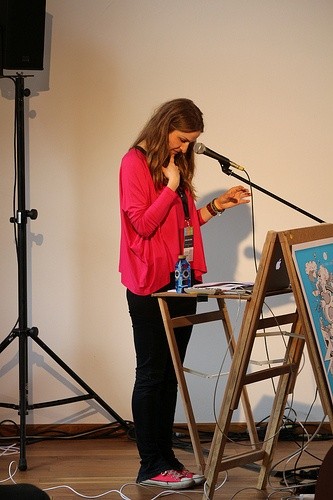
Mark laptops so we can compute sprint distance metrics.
[221,235,289,294]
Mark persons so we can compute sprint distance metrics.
[118,98,252,489]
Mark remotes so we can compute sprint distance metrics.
[184,288,222,294]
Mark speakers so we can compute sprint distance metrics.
[0,0,46,70]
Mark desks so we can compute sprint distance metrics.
[149,286,297,500]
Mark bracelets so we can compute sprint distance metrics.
[212,198,225,216]
[206,203,217,216]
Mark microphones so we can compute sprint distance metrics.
[192,143,244,171]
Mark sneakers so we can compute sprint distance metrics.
[140,470,195,490]
[181,466,207,486]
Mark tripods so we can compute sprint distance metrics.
[0,69,135,471]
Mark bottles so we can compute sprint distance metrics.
[174,255,192,294]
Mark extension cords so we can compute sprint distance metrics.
[298,494,315,500]
